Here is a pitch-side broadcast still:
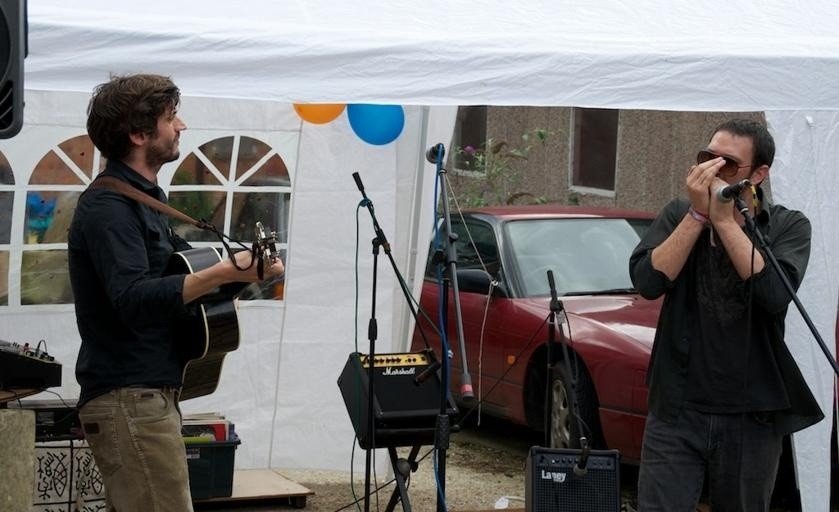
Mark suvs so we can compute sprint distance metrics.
[228,173,292,303]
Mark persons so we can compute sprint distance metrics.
[68,71,285,512]
[630,117,826,512]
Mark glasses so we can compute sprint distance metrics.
[697,150,759,177]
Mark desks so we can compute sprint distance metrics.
[191,467,316,511]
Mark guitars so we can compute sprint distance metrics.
[165,222,277,402]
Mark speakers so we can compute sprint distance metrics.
[337,351,460,450]
[525,446,621,512]
[0,0,29,139]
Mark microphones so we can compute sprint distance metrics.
[573,446,591,476]
[714,179,751,203]
[426,142,445,163]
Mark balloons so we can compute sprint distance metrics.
[347,101,405,146]
[292,103,345,124]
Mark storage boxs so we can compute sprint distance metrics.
[180,429,241,500]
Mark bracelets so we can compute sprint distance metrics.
[688,204,717,248]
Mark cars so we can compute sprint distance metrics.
[409,206,666,466]
